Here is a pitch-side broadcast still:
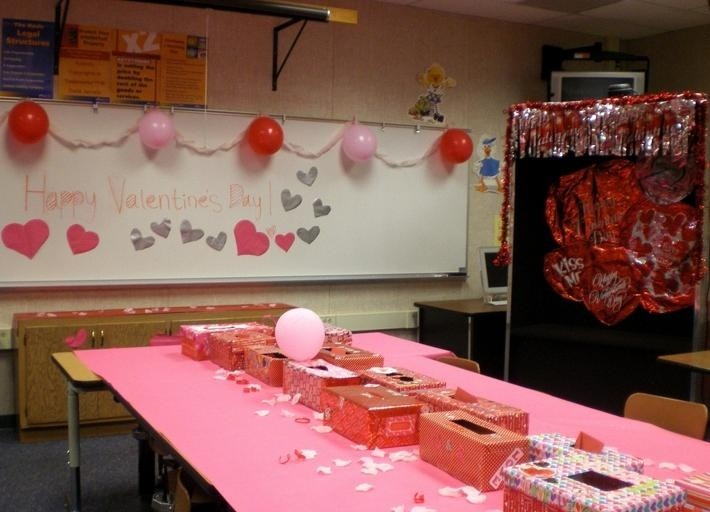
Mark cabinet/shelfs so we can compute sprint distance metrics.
[15,310,282,430]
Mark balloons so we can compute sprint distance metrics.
[275,308,324,361]
[246,116,283,157]
[439,129,474,163]
[9,100,48,144]
[138,109,175,149]
[343,124,376,161]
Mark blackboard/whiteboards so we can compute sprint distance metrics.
[0,97,470,289]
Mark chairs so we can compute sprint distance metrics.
[623,393,709,440]
[170,467,192,512]
[438,357,480,375]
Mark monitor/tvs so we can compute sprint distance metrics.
[480,246,509,305]
[550,71,645,102]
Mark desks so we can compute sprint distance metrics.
[413,297,508,360]
[49,331,710,512]
[655,349,709,375]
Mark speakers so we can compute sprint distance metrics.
[542,45,562,80]
